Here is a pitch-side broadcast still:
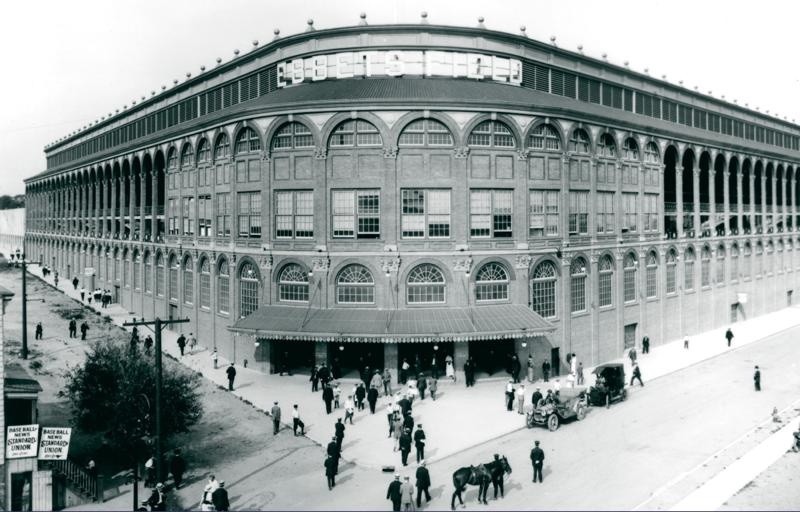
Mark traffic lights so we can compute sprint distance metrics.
[139,444,158,474]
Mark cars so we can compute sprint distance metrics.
[583,362,628,409]
[527,384,588,429]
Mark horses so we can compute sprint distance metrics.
[451,455,512,510]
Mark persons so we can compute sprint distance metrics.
[628,335,650,387]
[683,334,689,349]
[463,354,583,417]
[142,449,230,512]
[492,454,505,501]
[530,441,545,483]
[213,349,219,369]
[226,362,237,391]
[33,266,112,343]
[754,365,761,391]
[724,327,734,347]
[130,325,198,358]
[271,355,454,512]
